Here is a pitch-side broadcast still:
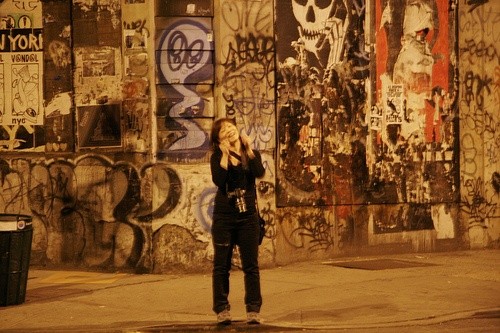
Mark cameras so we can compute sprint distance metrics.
[227,188,247,213]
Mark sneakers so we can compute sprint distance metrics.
[217,310,231,325]
[246,312,260,324]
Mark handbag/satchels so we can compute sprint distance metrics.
[258,210,266,245]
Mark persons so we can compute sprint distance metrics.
[209,118,268,324]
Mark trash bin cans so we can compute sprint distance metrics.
[0,213,34,306]
